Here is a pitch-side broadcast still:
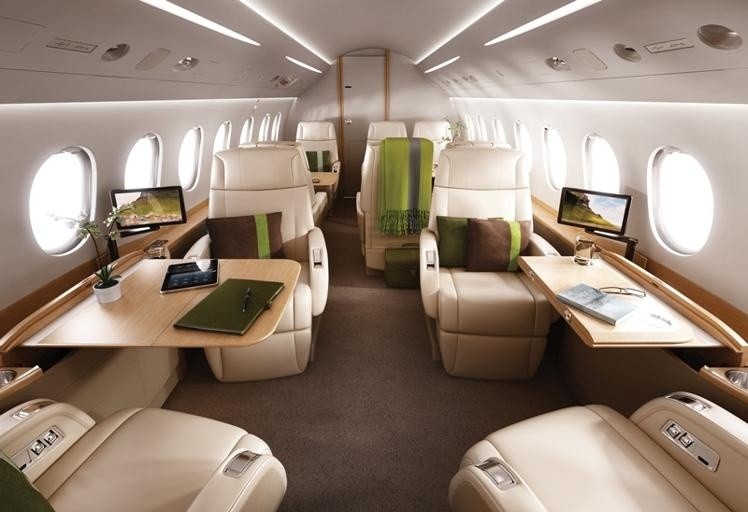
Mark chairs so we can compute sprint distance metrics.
[420,150,561,384]
[448,392,748,512]
[184,148,329,383]
[356,118,512,277]
[0,399,287,512]
[238,120,341,222]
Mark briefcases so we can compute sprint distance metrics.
[383,243,419,288]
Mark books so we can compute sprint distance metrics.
[555,281,643,327]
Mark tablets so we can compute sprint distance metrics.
[160,258,218,293]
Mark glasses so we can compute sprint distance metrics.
[599,287,646,298]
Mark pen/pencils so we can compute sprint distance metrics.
[242,288,251,313]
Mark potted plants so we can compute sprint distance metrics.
[50,200,136,304]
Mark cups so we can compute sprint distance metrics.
[574,231,595,266]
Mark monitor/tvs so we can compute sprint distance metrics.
[109,187,188,230]
[556,188,634,235]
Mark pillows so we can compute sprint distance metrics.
[436,215,471,269]
[466,218,531,272]
[0,458,55,512]
[307,150,328,173]
[205,211,286,259]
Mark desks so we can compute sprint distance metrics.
[518,254,723,349]
[22,258,301,345]
